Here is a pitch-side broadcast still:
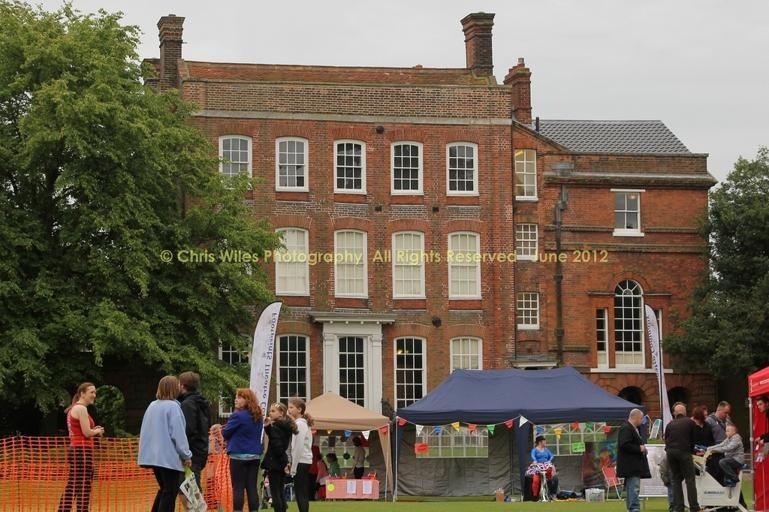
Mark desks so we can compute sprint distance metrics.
[326,479,378,501]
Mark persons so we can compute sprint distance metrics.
[351,437,366,479]
[659,400,745,510]
[753,395,769,446]
[57,382,105,512]
[221,387,263,511]
[262,402,300,512]
[203,424,225,509]
[530,435,559,500]
[260,452,341,509]
[288,397,314,512]
[137,374,193,512]
[614,408,652,512]
[178,370,211,494]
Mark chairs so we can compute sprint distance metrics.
[602,466,626,501]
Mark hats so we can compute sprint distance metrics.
[536,436,545,442]
